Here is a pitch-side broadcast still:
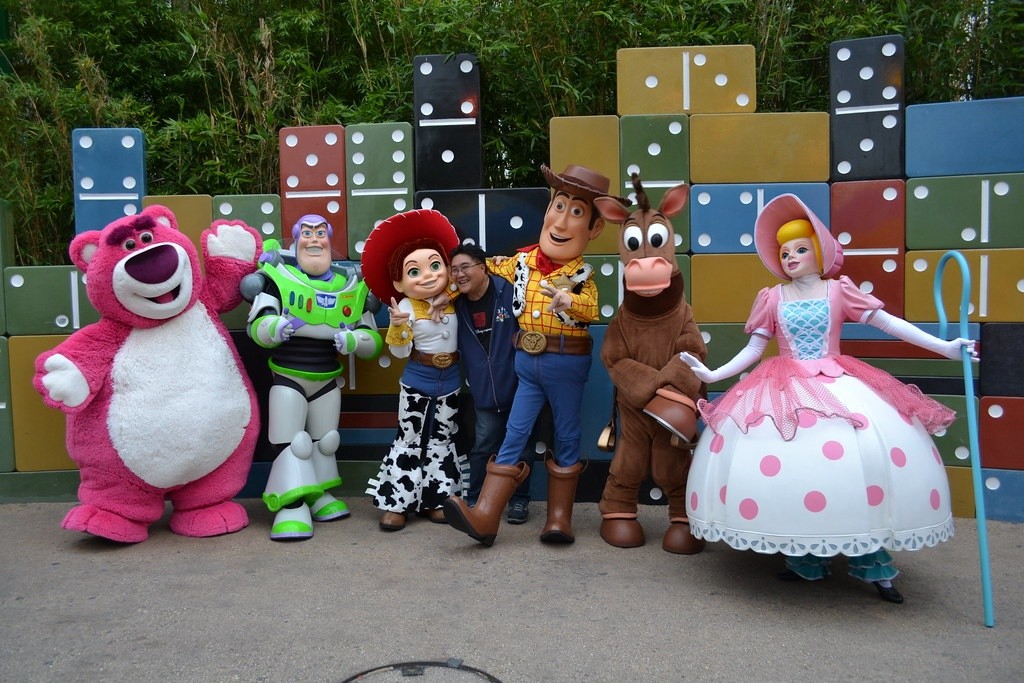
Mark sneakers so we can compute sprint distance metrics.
[468,504,474,510]
[507,500,529,524]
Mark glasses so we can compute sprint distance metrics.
[449,262,483,277]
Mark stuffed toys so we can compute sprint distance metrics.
[593,173,709,554]
[33,205,263,541]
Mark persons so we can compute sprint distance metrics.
[678,192,980,602]
[361,209,463,530]
[248,213,384,539]
[445,163,631,545]
[449,244,535,524]
